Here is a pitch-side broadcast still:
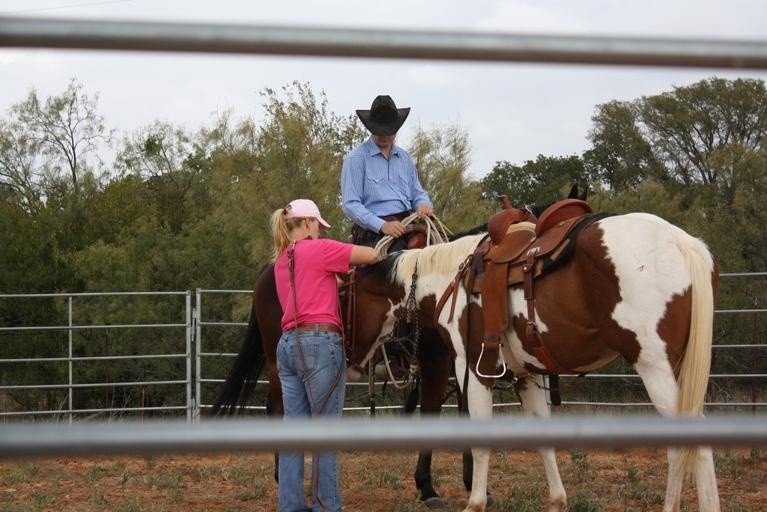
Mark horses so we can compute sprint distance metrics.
[336,210,723,512]
[204,181,592,512]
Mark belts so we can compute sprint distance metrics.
[291,322,341,334]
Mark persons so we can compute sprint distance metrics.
[340,94,435,379]
[269,197,380,512]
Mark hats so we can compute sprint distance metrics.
[283,199,332,230]
[356,96,411,137]
